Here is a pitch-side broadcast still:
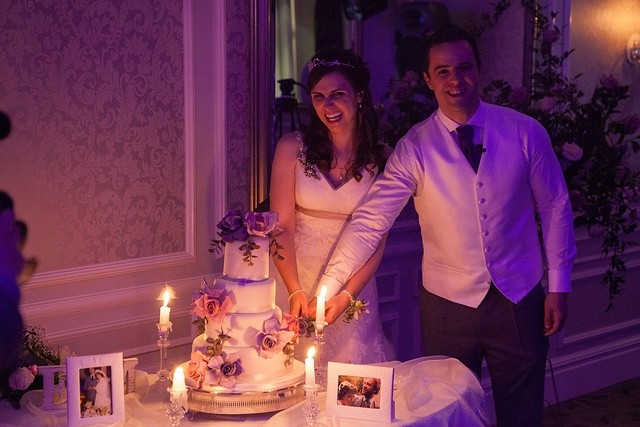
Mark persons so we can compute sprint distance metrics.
[336,379,357,408]
[357,378,379,407]
[266,49,397,376]
[91,370,110,410]
[317,25,574,426]
[83,368,97,403]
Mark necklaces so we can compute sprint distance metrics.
[330,143,355,182]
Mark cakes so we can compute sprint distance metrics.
[183,208,319,392]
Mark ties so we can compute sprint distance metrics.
[456,123,482,173]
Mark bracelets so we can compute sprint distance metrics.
[342,290,360,309]
[286,289,307,300]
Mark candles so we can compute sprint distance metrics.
[316,296,325,329]
[160,306,170,332]
[171,371,182,399]
[305,357,315,395]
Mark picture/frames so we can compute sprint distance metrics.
[65,351,126,427]
[325,361,394,423]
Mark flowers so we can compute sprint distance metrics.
[493,52,638,313]
[188,277,236,323]
[367,67,438,148]
[185,332,243,395]
[346,299,370,327]
[245,315,311,361]
[5,321,75,411]
[207,201,286,262]
